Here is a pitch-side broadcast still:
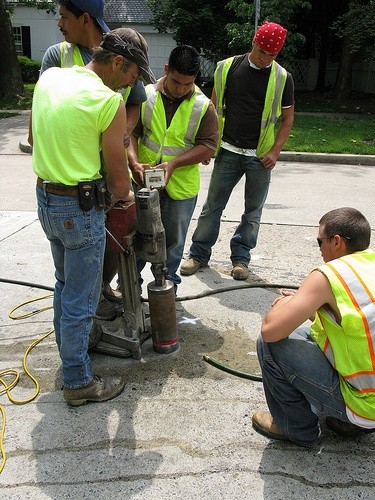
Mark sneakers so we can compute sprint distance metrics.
[231,263,249,280]
[180,255,208,275]
[252,411,321,449]
[325,416,375,438]
[88,324,103,350]
[102,284,121,304]
[63,371,125,407]
[94,294,118,322]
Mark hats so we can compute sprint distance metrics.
[99,27,157,84]
[59,0,111,33]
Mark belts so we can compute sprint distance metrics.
[37,177,80,197]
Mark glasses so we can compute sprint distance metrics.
[316,236,351,247]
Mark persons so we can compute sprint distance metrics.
[39,0,147,320]
[252,207,375,446]
[30,28,156,404]
[117,45,218,297]
[181,20,294,281]
